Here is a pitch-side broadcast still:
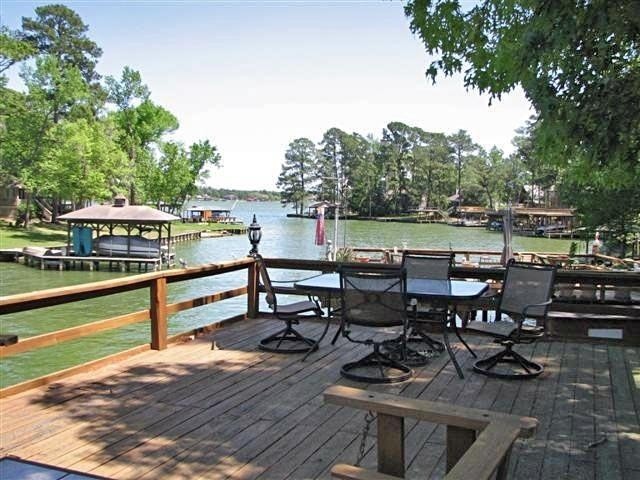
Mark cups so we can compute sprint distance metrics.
[632,236,639,258]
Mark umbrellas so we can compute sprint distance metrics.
[498,207,515,281]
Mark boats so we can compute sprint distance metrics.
[93,233,177,262]
[408,204,596,238]
[183,205,243,224]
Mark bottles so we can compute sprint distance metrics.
[592,230,600,255]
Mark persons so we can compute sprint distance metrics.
[325,240,334,262]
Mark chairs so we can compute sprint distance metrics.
[252,252,322,354]
[381,249,456,356]
[466,257,562,380]
[339,261,417,385]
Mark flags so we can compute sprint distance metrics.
[313,207,326,247]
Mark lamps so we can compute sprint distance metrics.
[245,213,263,257]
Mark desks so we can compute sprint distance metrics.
[293,273,488,369]
